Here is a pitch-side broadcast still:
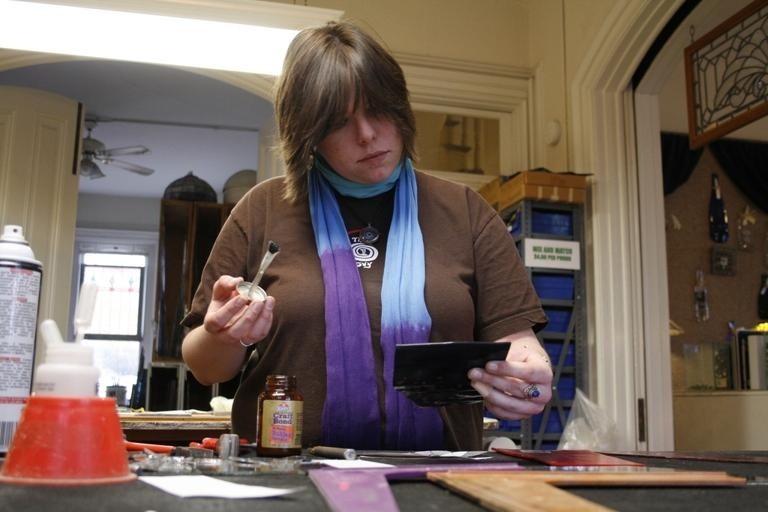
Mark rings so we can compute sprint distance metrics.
[239,339,254,348]
[520,382,541,400]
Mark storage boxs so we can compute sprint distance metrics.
[476,170,589,212]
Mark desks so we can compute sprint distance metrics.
[0,449,768,511]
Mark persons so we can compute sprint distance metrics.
[176,22,555,451]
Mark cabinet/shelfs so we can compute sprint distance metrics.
[483,201,593,452]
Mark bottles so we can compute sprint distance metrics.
[256,373,304,457]
[31,342,100,398]
[709,173,729,242]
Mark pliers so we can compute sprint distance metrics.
[122,437,248,459]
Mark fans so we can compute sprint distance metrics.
[81,113,155,180]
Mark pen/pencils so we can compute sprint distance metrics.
[307,446,356,461]
